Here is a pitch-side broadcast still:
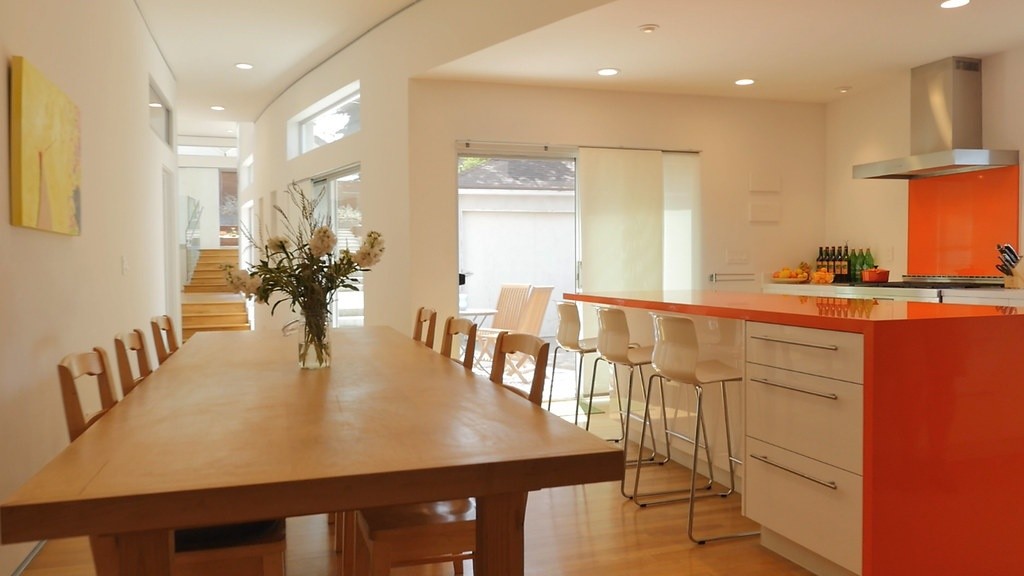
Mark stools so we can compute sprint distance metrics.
[632,312,761,545]
[546,300,657,463]
[585,306,715,499]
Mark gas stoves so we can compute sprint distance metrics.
[853,273,1006,289]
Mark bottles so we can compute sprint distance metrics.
[849,247,875,282]
[816,246,849,282]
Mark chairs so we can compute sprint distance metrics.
[327,307,551,576]
[473,285,556,382]
[474,285,533,366]
[56,315,288,576]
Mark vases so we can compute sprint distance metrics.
[298,308,333,370]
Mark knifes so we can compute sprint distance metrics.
[995,243,1021,277]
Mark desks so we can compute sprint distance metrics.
[459,309,496,375]
[0,325,626,576]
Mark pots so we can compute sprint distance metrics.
[860,265,890,283]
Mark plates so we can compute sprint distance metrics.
[771,277,807,284]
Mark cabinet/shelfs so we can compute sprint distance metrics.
[740,321,863,576]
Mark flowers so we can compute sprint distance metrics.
[215,180,385,365]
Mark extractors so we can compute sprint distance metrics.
[851,56,1019,179]
